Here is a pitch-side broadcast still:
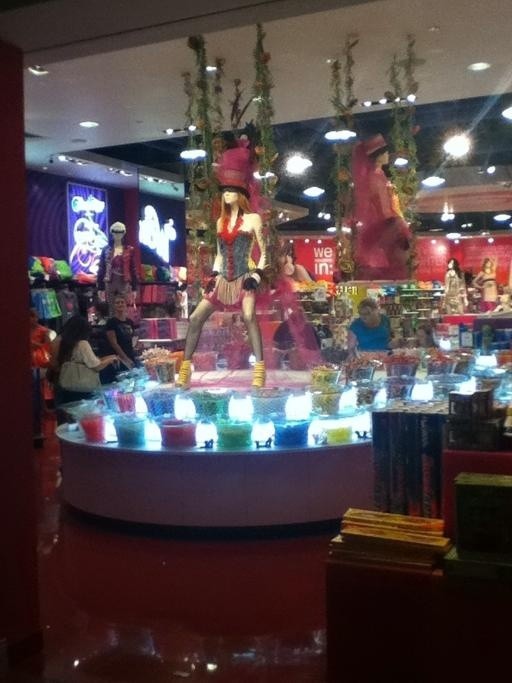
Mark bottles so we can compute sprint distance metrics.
[480,324,493,353]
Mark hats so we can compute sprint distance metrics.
[217,169,251,199]
[364,132,389,156]
[110,221,126,231]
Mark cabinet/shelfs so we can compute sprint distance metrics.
[296,288,336,327]
[29,271,91,448]
[379,285,447,347]
[322,533,457,682]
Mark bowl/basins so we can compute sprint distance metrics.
[79,349,472,448]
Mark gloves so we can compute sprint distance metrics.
[243,268,265,293]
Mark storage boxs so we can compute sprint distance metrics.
[438,424,512,538]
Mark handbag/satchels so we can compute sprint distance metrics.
[57,339,102,394]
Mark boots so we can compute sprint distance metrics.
[250,360,266,390]
[174,359,193,392]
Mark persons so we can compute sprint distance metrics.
[30,309,51,448]
[347,297,393,360]
[441,259,468,315]
[273,307,321,370]
[177,186,266,386]
[270,241,315,292]
[473,259,497,312]
[350,131,413,281]
[54,315,115,425]
[99,294,139,384]
[98,220,144,319]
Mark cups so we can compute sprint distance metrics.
[487,327,511,351]
